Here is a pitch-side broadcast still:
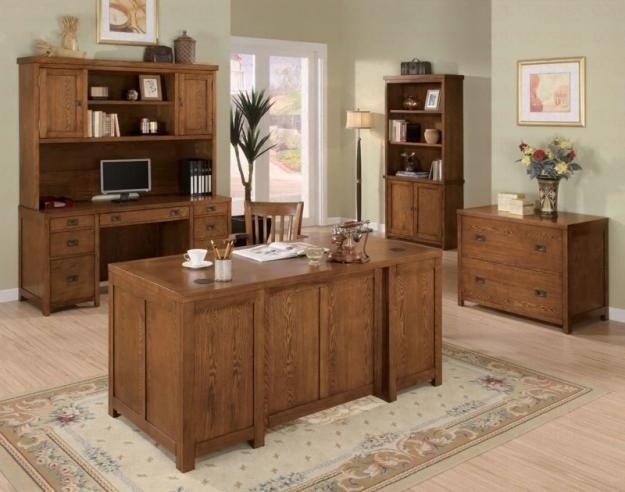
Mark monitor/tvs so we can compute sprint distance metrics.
[100,159,152,203]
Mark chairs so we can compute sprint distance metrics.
[241,198,306,249]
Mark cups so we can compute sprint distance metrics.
[306,245,324,266]
[63,33,77,50]
[214,257,233,282]
[139,117,159,135]
[183,249,208,264]
[425,128,440,145]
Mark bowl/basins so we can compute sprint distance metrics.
[56,48,86,59]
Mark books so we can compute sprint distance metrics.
[387,119,420,144]
[427,158,443,181]
[176,157,212,196]
[232,237,331,262]
[86,109,122,139]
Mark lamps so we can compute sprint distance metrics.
[345,105,372,224]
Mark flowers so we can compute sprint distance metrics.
[509,133,586,182]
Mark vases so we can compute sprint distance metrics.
[534,172,565,220]
[422,127,440,146]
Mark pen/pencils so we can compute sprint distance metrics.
[210,239,234,259]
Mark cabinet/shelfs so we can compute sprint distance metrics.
[157,188,233,260]
[382,174,466,251]
[15,204,96,320]
[379,74,466,185]
[13,51,220,213]
[454,200,612,336]
[92,194,191,230]
[98,225,443,473]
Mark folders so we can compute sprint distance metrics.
[177,158,212,196]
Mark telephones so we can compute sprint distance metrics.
[326,219,373,264]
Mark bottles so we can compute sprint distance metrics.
[174,31,196,65]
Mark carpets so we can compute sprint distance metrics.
[2,323,615,492]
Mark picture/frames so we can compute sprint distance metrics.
[422,87,441,111]
[515,56,586,127]
[94,0,160,48]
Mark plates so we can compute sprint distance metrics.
[181,261,213,269]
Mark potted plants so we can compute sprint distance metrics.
[225,81,280,248]
